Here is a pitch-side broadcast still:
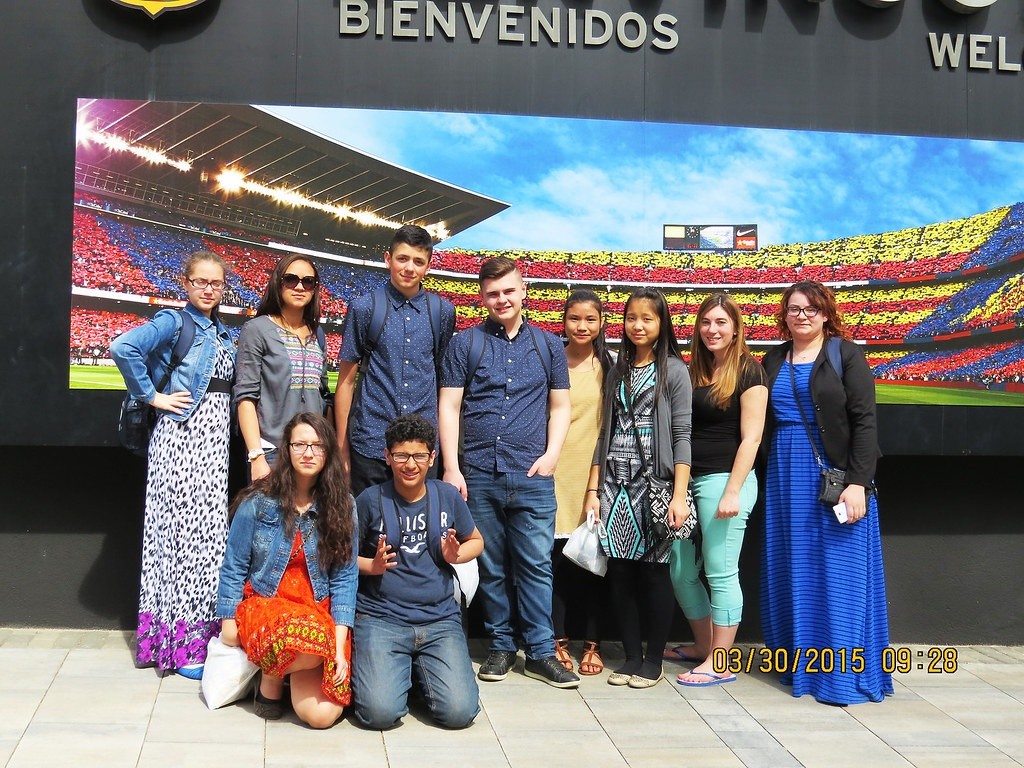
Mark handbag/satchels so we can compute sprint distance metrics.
[201,632,261,711]
[646,474,698,540]
[562,509,608,577]
[818,468,868,514]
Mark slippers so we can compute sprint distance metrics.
[677,667,737,686]
[663,646,705,662]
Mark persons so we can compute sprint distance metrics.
[664,293,770,687]
[110,250,238,679]
[69,206,1024,389]
[351,414,485,728]
[439,257,581,688]
[584,286,693,688]
[756,279,894,704]
[551,290,618,675]
[215,412,359,729]
[233,253,331,486]
[334,224,456,498]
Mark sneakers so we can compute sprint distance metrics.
[524,656,581,687]
[478,650,518,680]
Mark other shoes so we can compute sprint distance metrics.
[253,679,286,720]
[175,666,203,680]
[629,666,664,688]
[608,672,631,685]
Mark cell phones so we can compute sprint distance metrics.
[833,502,848,524]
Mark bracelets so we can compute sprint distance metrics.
[587,489,598,492]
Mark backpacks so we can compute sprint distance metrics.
[118,309,196,457]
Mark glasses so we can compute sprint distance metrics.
[281,273,317,291]
[186,275,226,291]
[289,443,328,456]
[786,306,822,317]
[390,452,432,463]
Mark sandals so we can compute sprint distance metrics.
[578,640,604,675]
[555,638,573,671]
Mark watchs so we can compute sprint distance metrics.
[248,449,264,462]
